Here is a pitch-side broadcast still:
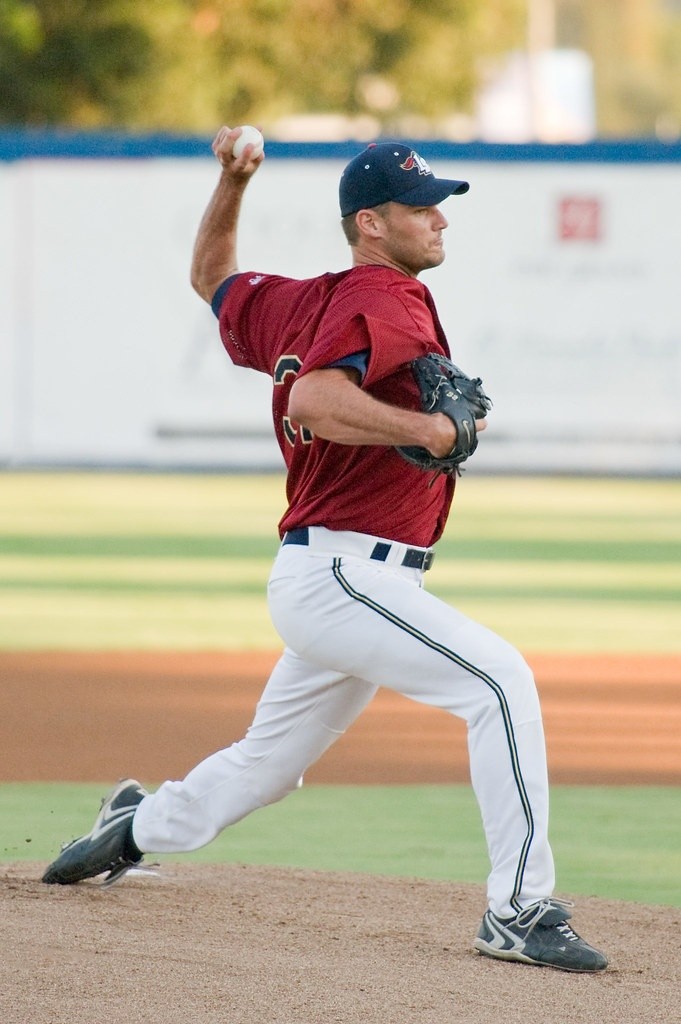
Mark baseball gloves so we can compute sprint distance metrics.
[394,351,491,469]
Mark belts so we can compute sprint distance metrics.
[285,528,436,573]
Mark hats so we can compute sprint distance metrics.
[340,143,469,211]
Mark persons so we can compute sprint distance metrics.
[41,126,610,974]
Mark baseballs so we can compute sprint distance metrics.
[232,123,265,161]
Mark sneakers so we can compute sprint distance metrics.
[473,897,609,975]
[43,777,149,884]
